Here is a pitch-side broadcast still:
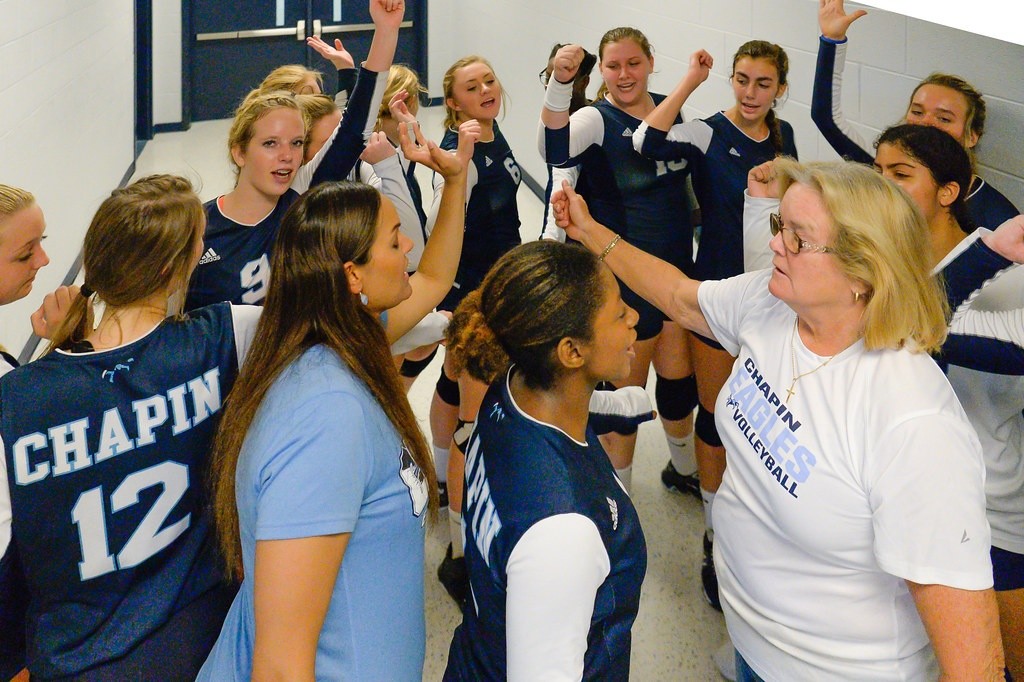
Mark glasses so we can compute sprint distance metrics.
[539,68,549,87]
[770,212,836,255]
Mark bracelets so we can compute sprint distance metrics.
[598,234,622,261]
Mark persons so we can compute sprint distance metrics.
[192,125,467,682]
[186,0,799,614]
[550,157,1005,682]
[0,185,94,382]
[436,239,657,681]
[811,0,1024,680]
[0,175,262,682]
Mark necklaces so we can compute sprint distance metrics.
[784,318,861,404]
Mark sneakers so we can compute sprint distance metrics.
[661,459,702,500]
[700,531,723,612]
[437,541,466,613]
[428,481,449,511]
